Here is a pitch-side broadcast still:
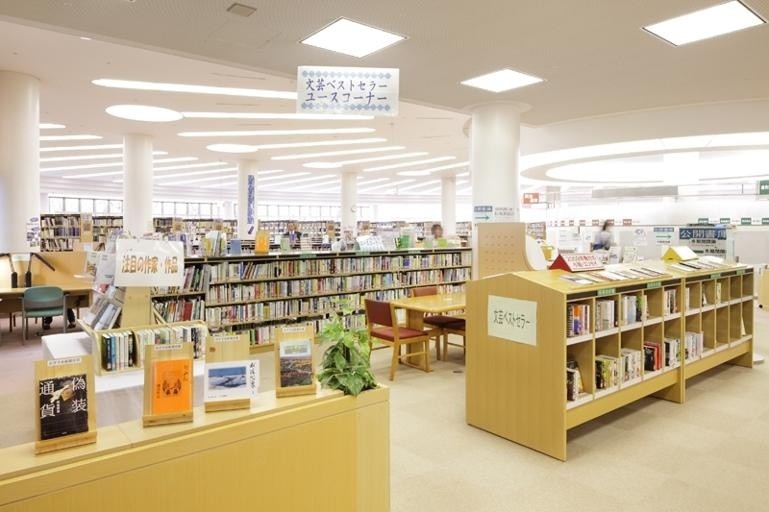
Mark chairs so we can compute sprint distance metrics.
[17,285,67,346]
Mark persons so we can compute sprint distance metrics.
[282,222,301,248]
[592,223,613,251]
[423,224,447,248]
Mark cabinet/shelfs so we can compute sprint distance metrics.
[463,255,755,462]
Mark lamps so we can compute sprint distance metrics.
[25,252,57,288]
[0,253,18,289]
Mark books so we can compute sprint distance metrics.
[154,217,236,239]
[567,281,721,403]
[91,251,473,371]
[93,219,123,241]
[41,216,80,251]
